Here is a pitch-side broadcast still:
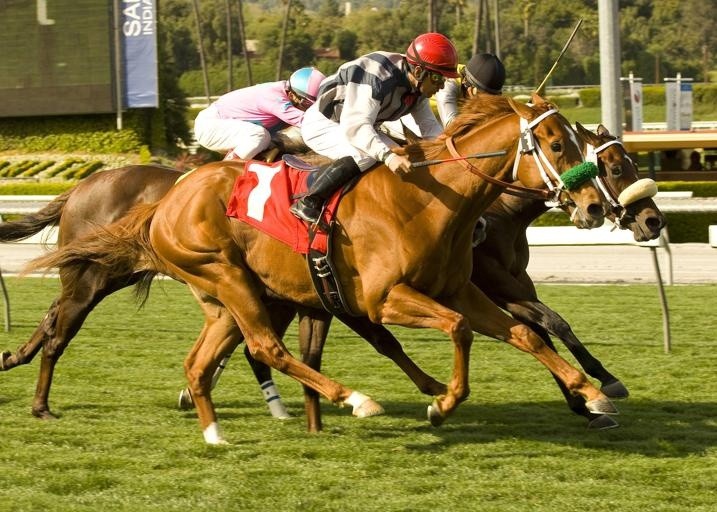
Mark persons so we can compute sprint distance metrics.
[287,32,461,228]
[379,51,506,144]
[192,66,325,164]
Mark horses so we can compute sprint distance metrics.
[0,161,486,420]
[15,91,604,446]
[178,121,665,432]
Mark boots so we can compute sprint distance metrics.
[289,156,361,223]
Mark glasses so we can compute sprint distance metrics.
[465,77,484,96]
[413,42,447,85]
[288,76,311,111]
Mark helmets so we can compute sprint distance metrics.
[405,33,461,78]
[464,53,506,96]
[285,66,326,103]
